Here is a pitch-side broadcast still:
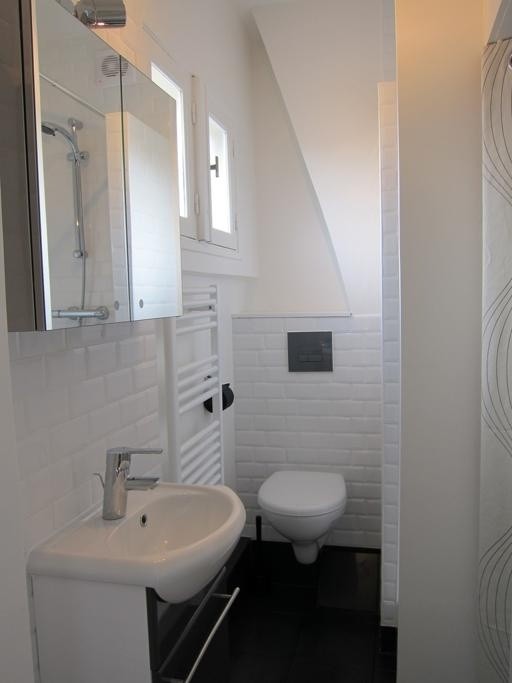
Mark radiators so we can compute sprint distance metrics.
[164,281,229,486]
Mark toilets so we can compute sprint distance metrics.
[257,470,347,563]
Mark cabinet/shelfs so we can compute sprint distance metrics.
[28,2,186,330]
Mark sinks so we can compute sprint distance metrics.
[28,482,247,602]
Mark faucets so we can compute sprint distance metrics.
[102,445,160,521]
